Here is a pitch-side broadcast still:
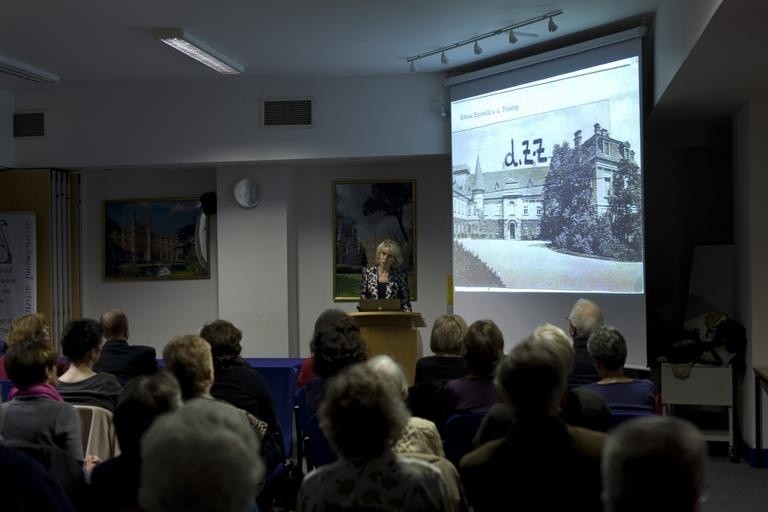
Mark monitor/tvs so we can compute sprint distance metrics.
[359,298,401,311]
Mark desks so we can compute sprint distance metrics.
[154,357,305,456]
[755,366,768,469]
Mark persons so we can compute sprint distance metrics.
[0,308,717,512]
[359,239,413,312]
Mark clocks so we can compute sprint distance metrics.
[233,177,264,209]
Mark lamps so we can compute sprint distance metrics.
[159,30,245,76]
[408,8,565,73]
[0,56,60,84]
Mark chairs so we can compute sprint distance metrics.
[70,403,122,486]
[0,436,86,512]
[440,409,488,464]
[398,451,469,512]
[611,410,658,425]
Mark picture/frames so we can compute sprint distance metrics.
[331,179,418,301]
[101,195,212,282]
